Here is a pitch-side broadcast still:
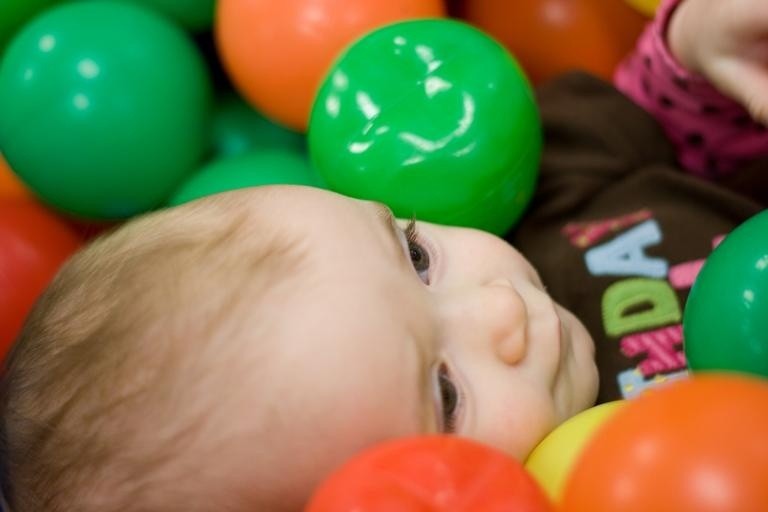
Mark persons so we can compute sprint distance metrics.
[0,0,768,511]
